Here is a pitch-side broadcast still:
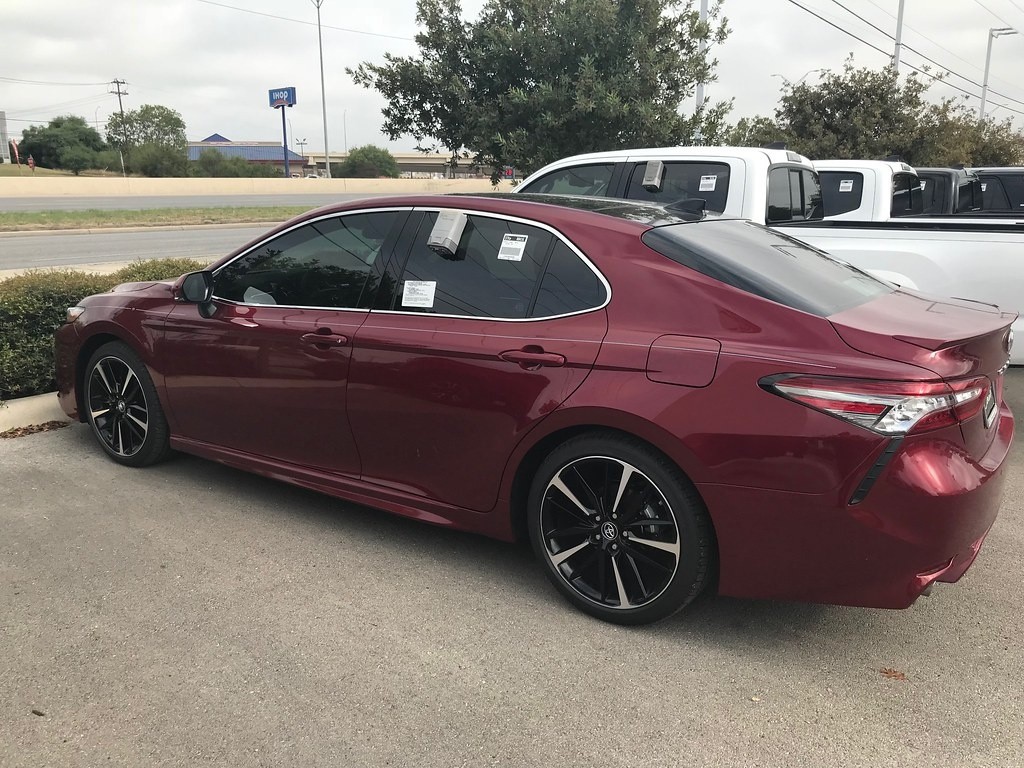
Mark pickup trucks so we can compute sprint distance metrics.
[509,146,1024,372]
[811,160,1024,225]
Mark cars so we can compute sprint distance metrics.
[49,192,1019,628]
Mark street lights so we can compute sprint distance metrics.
[296,138,308,178]
